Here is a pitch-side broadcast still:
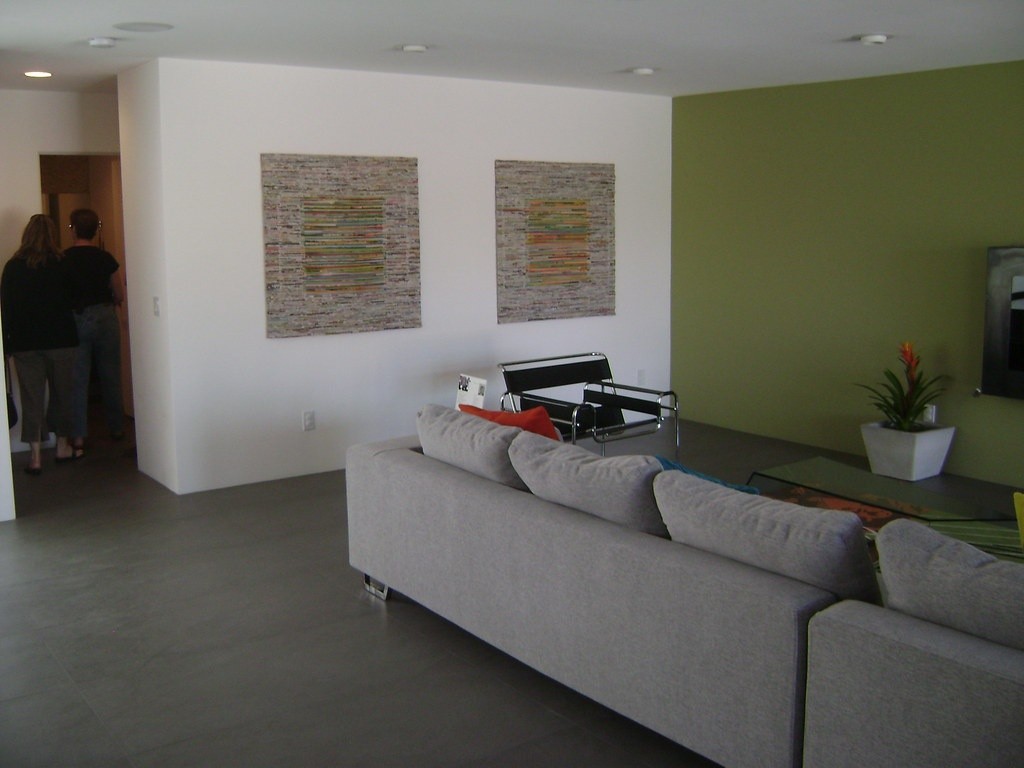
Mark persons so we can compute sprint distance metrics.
[60,210,123,458]
[1,214,85,473]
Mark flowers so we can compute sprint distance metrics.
[853,341,952,429]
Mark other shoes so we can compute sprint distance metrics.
[111,431,126,439]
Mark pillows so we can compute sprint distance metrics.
[874,514,1024,651]
[413,406,525,493]
[506,429,665,540]
[653,466,878,605]
[459,403,557,443]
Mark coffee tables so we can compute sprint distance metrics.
[749,453,1024,565]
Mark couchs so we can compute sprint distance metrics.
[345,403,1024,768]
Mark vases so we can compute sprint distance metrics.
[860,422,956,481]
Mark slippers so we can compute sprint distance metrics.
[55,447,85,462]
[24,464,41,476]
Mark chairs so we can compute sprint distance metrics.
[501,352,681,455]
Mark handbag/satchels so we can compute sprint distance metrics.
[3,356,18,429]
